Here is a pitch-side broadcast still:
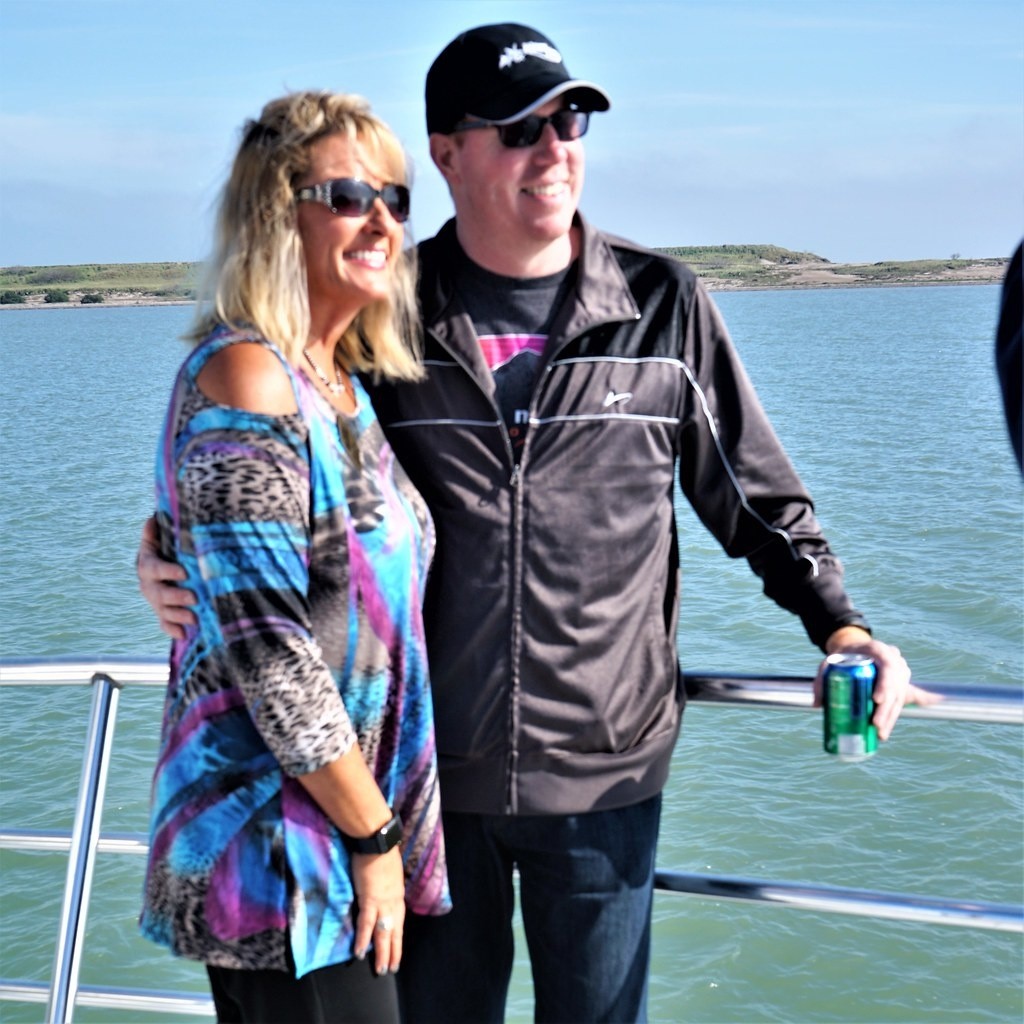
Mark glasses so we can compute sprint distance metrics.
[291,177,411,224]
[439,106,592,149]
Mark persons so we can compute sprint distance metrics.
[995,235,1023,476]
[135,23,946,1024]
[135,89,455,1024]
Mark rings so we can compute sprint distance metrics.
[378,919,396,930]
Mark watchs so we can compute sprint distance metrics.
[334,807,405,855]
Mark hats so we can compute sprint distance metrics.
[424,23,610,138]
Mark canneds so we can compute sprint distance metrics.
[821,652,878,763]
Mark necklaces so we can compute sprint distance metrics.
[301,346,346,396]
